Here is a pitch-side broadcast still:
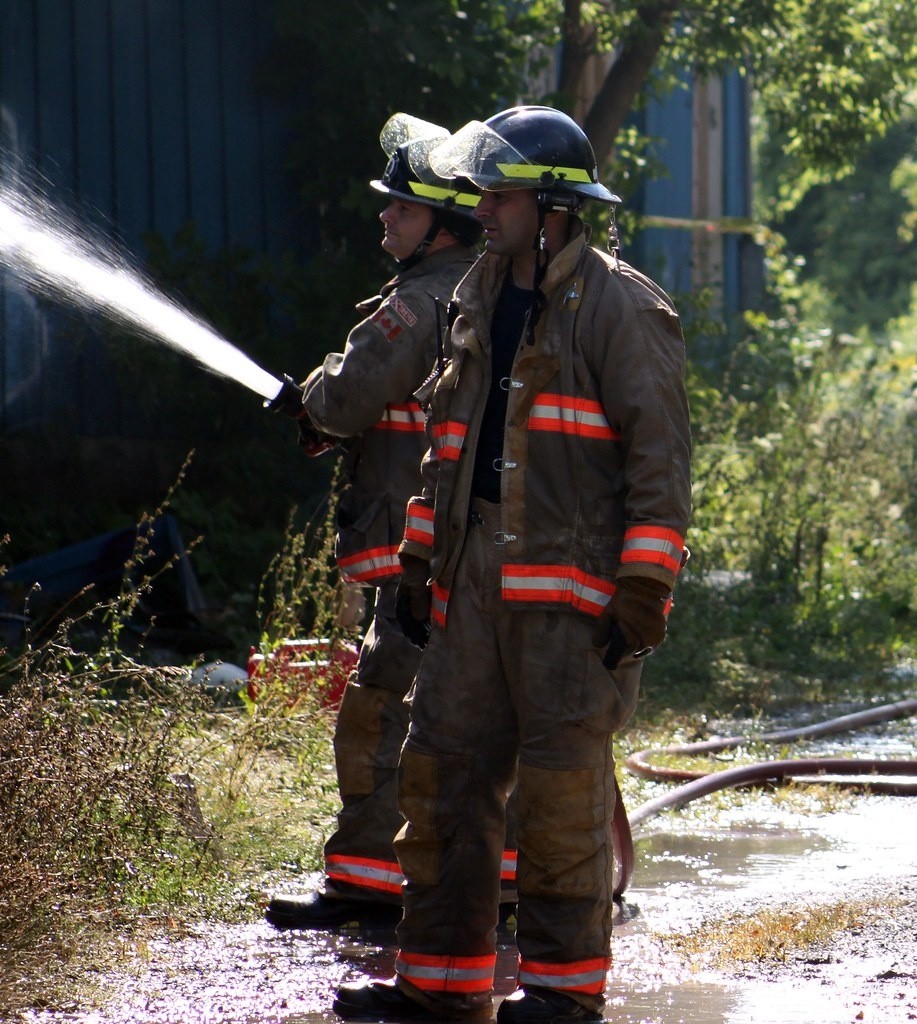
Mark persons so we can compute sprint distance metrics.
[331,103,696,1023]
[262,132,521,932]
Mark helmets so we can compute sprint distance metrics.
[453,105,622,204]
[370,136,483,222]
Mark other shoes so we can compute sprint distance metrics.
[496,983,605,1024]
[264,875,405,931]
[332,974,493,1024]
[495,902,518,945]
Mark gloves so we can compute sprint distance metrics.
[591,576,672,671]
[394,554,435,650]
